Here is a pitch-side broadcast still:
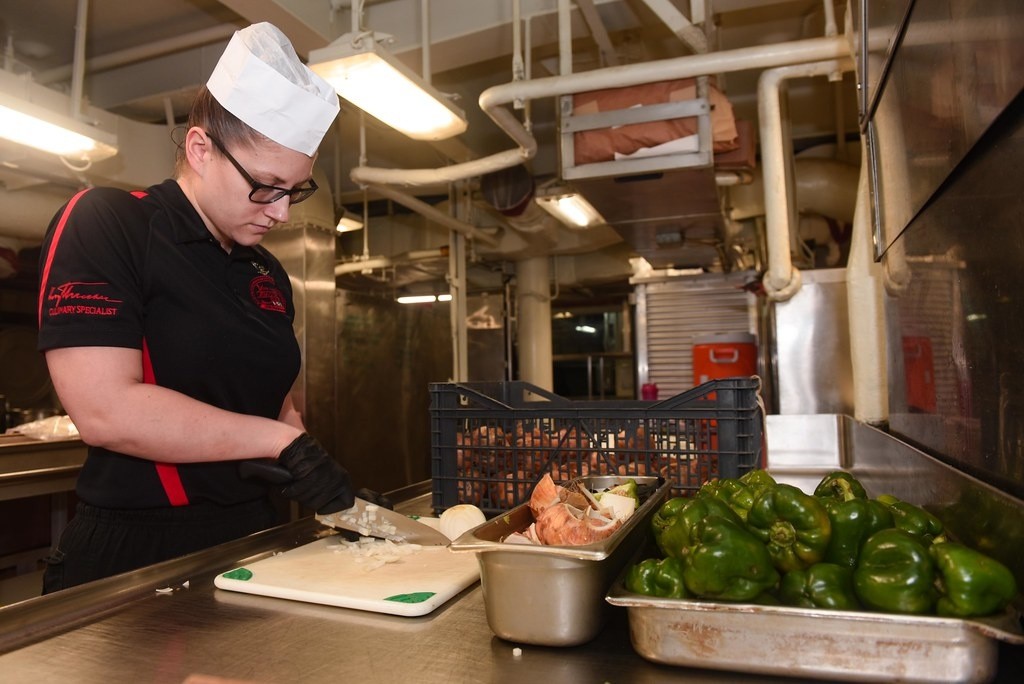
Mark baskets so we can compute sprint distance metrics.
[428,376,762,520]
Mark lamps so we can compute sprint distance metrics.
[535,95,607,232]
[0,0,118,171]
[302,0,471,144]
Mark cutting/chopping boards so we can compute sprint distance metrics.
[212,516,480,617]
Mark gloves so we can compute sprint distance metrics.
[277,434,355,515]
[334,487,393,542]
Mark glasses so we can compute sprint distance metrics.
[204,132,319,204]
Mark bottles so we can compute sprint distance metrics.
[0,394,61,434]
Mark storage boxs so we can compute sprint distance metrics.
[691,334,757,382]
[432,380,761,516]
[904,330,937,414]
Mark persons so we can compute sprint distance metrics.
[30,20,399,597]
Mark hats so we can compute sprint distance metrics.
[206,22,341,158]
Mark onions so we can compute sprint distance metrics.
[439,504,485,538]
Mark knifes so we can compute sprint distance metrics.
[247,459,452,547]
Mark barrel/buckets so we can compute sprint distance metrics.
[690,331,759,460]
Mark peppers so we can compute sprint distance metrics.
[625,472,1017,615]
